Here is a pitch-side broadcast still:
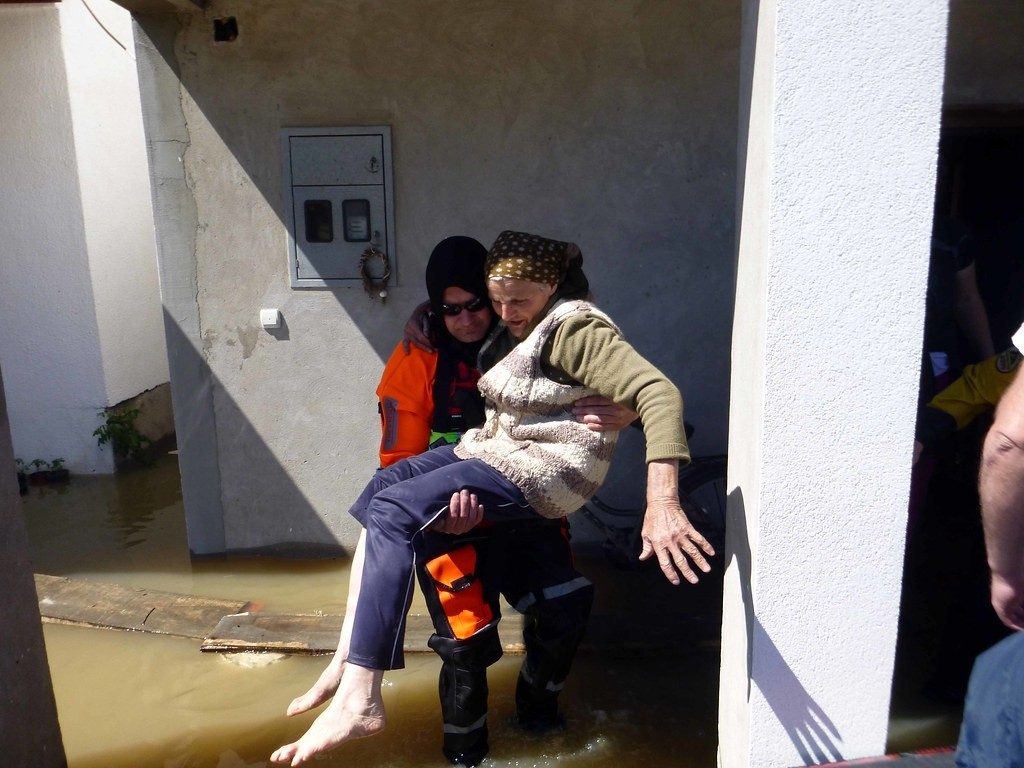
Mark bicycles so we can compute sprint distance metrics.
[570,417,729,618]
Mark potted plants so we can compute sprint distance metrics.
[15,458,70,495]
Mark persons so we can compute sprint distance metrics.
[375,231,646,768]
[909,161,1024,766]
[270,229,718,768]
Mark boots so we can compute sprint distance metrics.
[514,626,585,736]
[428,626,503,768]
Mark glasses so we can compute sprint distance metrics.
[440,297,489,317]
